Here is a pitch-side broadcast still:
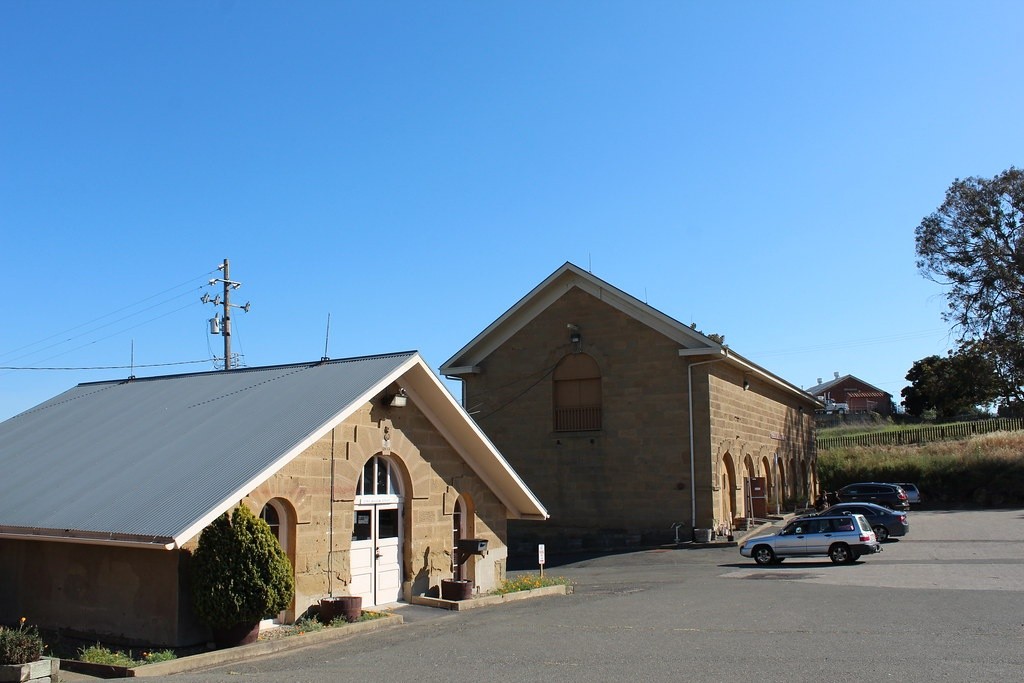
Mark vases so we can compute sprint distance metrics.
[695,528,712,542]
[0,657,61,683]
[735,517,750,531]
[441,577,471,600]
[321,596,362,623]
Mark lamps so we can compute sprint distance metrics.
[386,387,408,408]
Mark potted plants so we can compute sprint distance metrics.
[768,495,807,513]
[189,502,296,649]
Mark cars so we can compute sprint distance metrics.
[787,502,909,542]
[819,399,850,415]
[813,482,910,511]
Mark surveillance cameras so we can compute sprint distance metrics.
[567,323,580,331]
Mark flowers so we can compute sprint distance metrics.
[0,615,44,665]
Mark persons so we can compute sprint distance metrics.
[829,491,844,513]
[817,490,828,513]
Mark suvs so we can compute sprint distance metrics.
[739,512,883,566]
[889,483,922,508]
